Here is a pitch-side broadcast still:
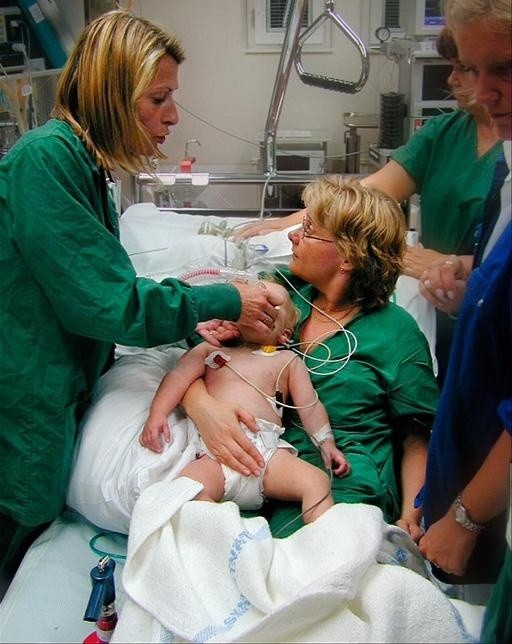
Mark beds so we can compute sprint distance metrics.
[1,201,440,643]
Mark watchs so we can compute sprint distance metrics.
[452,490,487,534]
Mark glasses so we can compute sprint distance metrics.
[300,216,333,243]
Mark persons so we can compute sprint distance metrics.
[138,280,352,527]
[412,0,512,608]
[238,172,442,547]
[231,24,507,389]
[0,9,285,606]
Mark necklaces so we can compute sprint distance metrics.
[311,302,356,323]
